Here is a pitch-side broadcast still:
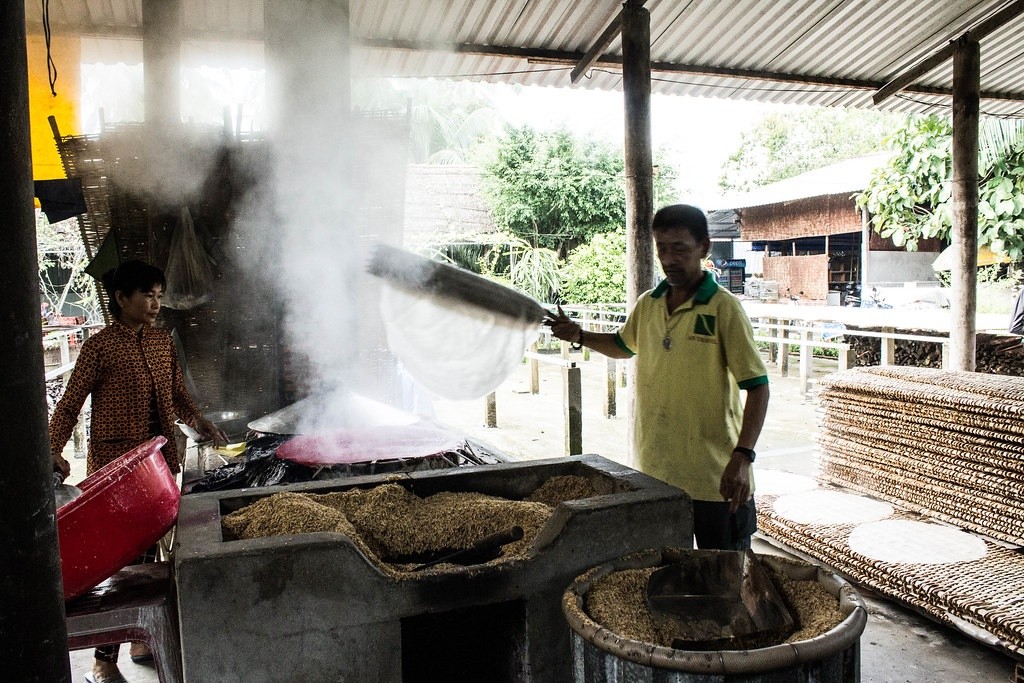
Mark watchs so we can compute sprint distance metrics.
[732,447,755,463]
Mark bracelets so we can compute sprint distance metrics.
[571,329,583,349]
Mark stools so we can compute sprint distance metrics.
[67,562,182,683]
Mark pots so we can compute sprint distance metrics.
[247,415,464,475]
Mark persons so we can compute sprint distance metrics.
[542,204,768,550]
[48,264,230,683]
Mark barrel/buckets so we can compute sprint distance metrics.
[561,548,869,683]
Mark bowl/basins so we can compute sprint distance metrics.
[174,410,255,443]
[56,435,181,604]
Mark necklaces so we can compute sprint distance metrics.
[662,308,683,351]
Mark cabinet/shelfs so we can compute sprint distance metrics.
[829,270,860,292]
